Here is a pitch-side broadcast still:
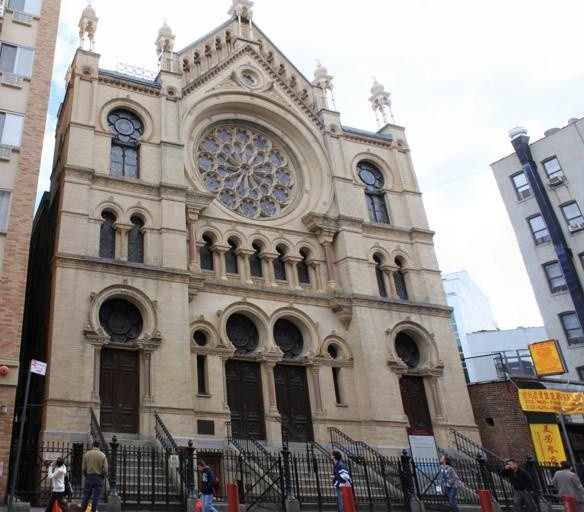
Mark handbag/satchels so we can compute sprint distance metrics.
[85,503,92,512]
[65,473,74,498]
[194,496,203,512]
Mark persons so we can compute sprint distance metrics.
[438,454,462,512]
[499,459,538,512]
[45,456,69,511]
[329,450,353,512]
[196,460,219,511]
[77,438,108,512]
[551,460,584,512]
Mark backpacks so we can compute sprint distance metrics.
[212,475,221,497]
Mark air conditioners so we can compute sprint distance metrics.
[549,176,562,186]
[568,222,581,232]
